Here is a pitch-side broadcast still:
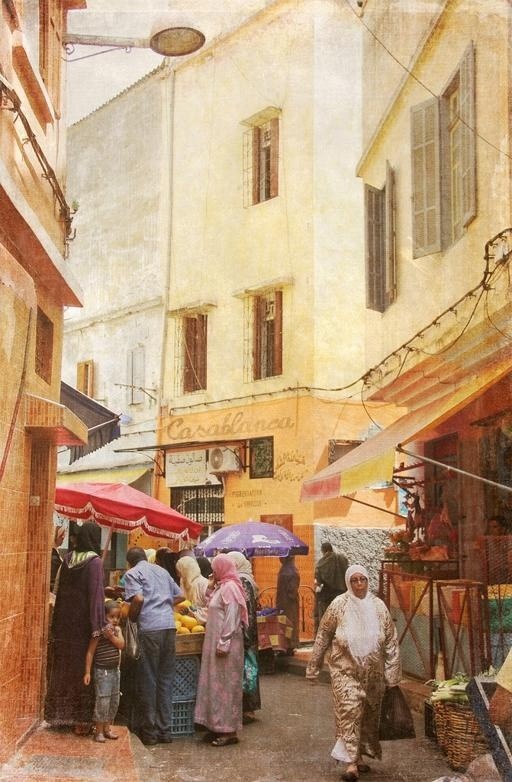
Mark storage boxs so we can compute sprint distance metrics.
[174,630,204,656]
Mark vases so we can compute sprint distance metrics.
[394,549,422,573]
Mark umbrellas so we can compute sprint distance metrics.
[192,516,310,564]
[54,481,205,565]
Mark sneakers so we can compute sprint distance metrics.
[343,765,370,782]
[94,731,118,743]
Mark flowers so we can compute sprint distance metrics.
[379,522,427,563]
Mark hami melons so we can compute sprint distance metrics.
[173,601,204,634]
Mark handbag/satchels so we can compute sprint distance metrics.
[377,685,416,739]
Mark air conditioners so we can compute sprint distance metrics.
[207,444,240,475]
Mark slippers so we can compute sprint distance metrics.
[212,738,238,746]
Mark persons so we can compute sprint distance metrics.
[305,564,402,781]
[488,648,511,731]
[314,542,349,631]
[275,556,300,657]
[43,521,262,748]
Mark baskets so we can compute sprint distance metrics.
[170,655,201,739]
[434,699,492,772]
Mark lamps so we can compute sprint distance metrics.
[60,0,207,57]
